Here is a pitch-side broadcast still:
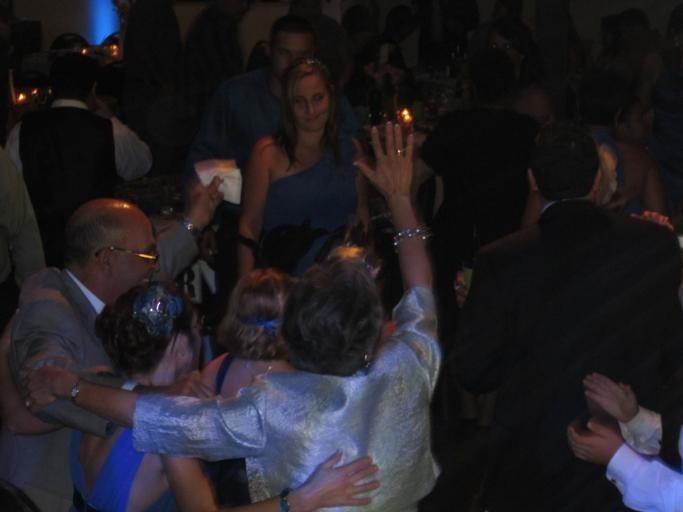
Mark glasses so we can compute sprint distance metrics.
[94,243,160,263]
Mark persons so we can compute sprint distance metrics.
[0,0,683,512]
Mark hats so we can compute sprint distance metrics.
[91,282,187,373]
